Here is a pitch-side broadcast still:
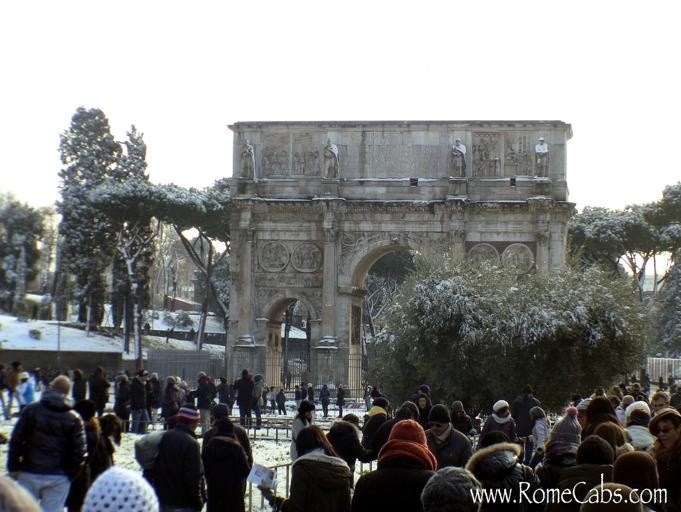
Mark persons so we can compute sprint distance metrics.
[0,360,680,512]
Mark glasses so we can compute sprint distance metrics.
[654,425,676,435]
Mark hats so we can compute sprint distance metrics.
[492,399,512,413]
[137,368,149,377]
[419,463,484,511]
[417,383,432,393]
[253,373,264,382]
[428,403,452,426]
[630,409,651,426]
[575,435,614,468]
[480,430,511,448]
[386,418,430,450]
[246,371,253,379]
[371,397,391,410]
[72,398,97,423]
[578,481,645,511]
[176,402,201,422]
[166,374,176,384]
[11,360,22,367]
[79,462,162,511]
[548,404,583,446]
[451,399,465,414]
[648,406,681,438]
[296,401,316,413]
[528,405,546,419]
